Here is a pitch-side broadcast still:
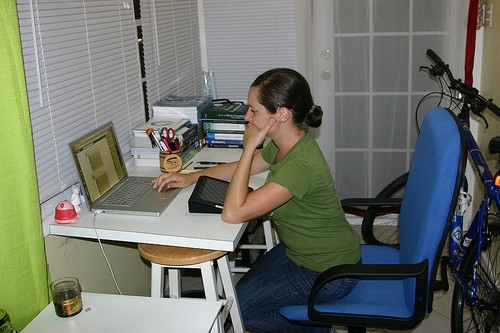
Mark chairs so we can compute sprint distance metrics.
[279,108,466,333]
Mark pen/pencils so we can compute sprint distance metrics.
[194,166,210,169]
[196,161,226,164]
[146,127,180,151]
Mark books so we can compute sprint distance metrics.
[131,96,263,167]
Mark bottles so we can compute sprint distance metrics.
[0,308,16,333]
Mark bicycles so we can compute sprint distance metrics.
[364,49,500,333]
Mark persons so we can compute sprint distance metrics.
[152,67,362,333]
[70,184,81,211]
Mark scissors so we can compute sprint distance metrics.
[162,127,175,143]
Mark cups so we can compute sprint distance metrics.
[160,151,183,173]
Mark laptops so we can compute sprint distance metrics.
[71,121,181,217]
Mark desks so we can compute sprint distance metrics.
[48,148,276,333]
[19,292,224,333]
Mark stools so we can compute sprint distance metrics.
[138,243,228,333]
